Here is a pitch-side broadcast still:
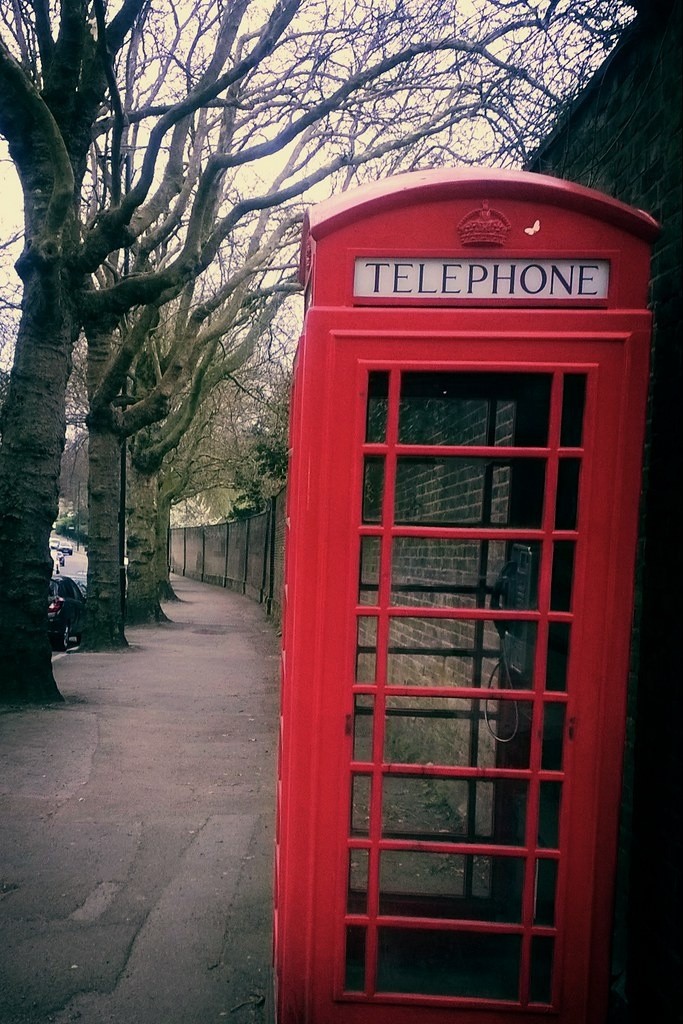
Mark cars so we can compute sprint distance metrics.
[50,534,74,574]
[47,574,87,651]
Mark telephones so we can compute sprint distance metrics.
[489,542,572,686]
